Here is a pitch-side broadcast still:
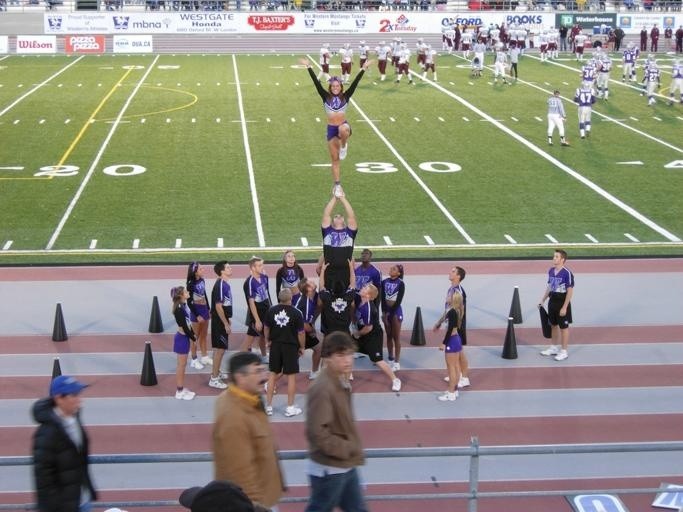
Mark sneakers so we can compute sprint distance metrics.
[560,140,569,145]
[218,370,228,380]
[264,383,277,393]
[539,345,558,356]
[208,375,228,389]
[264,402,273,416]
[190,358,203,370]
[259,355,270,365]
[338,142,349,160]
[316,78,322,83]
[308,370,321,381]
[444,375,451,383]
[176,388,196,400]
[202,355,212,365]
[284,406,303,417]
[396,78,401,84]
[333,185,344,197]
[458,377,471,388]
[502,79,507,83]
[438,390,460,401]
[549,141,554,145]
[345,80,350,85]
[341,80,344,86]
[407,79,413,85]
[494,78,498,84]
[391,378,402,392]
[555,349,569,361]
[581,134,585,140]
[386,360,401,373]
[586,129,590,137]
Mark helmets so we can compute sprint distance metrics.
[344,43,349,49]
[378,41,385,44]
[322,43,330,48]
[417,38,423,42]
[359,40,367,45]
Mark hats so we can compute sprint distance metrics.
[178,480,254,512]
[49,375,89,398]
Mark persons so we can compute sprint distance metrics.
[316,254,357,274]
[622,50,633,81]
[186,262,214,368]
[0,1,240,11]
[574,80,596,139]
[546,37,555,60]
[380,265,405,372]
[548,29,555,41]
[675,25,683,53]
[467,0,682,12]
[298,58,375,198]
[540,249,574,361]
[209,261,233,389]
[358,39,370,70]
[390,39,397,52]
[170,285,196,400]
[473,24,527,85]
[442,22,472,59]
[179,479,272,512]
[264,288,306,416]
[353,250,381,359]
[391,40,401,67]
[607,28,616,53]
[212,352,288,511]
[437,288,464,401]
[641,62,661,106]
[539,30,548,62]
[639,54,657,96]
[568,21,587,61]
[276,250,305,307]
[592,46,602,60]
[580,60,596,104]
[316,42,333,81]
[624,49,637,81]
[614,27,625,50]
[338,43,354,84]
[666,59,683,106]
[320,258,355,381]
[32,375,100,512]
[546,39,559,58]
[596,49,612,100]
[247,0,449,12]
[640,26,648,51]
[322,188,358,291]
[554,29,561,47]
[305,331,369,512]
[633,42,639,59]
[650,24,659,52]
[664,27,672,51]
[394,43,413,85]
[373,40,391,81]
[350,284,401,391]
[416,37,427,70]
[559,26,568,51]
[419,44,437,81]
[264,277,324,394]
[547,91,570,146]
[433,267,470,389]
[628,44,636,69]
[241,256,273,364]
